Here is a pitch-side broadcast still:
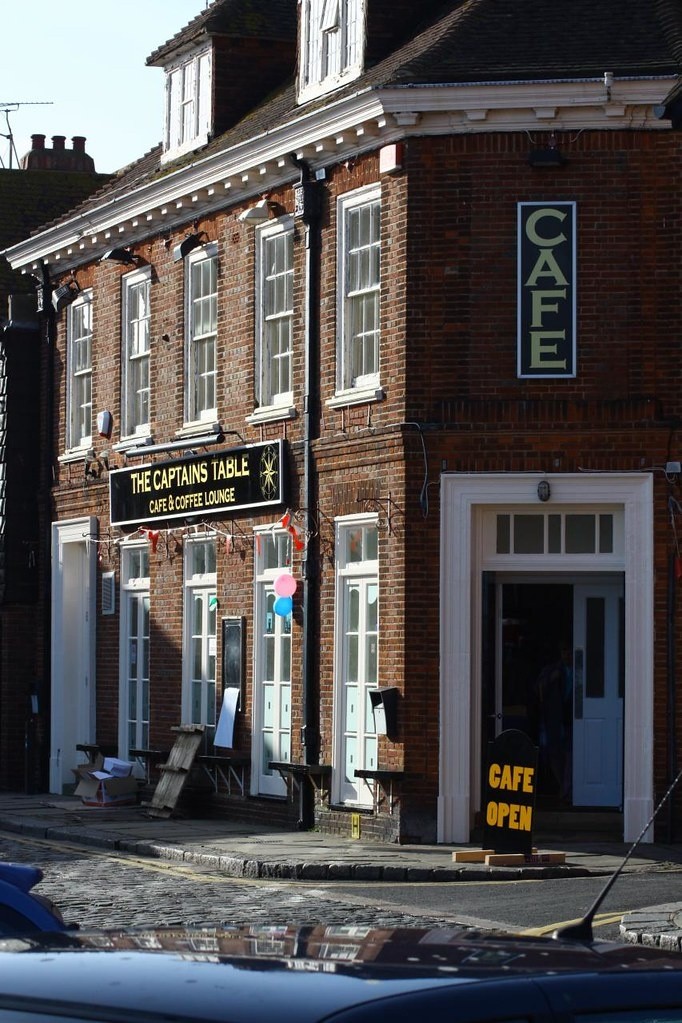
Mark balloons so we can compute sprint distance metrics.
[273,574,297,617]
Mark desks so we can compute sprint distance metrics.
[75,744,109,762]
[197,755,250,797]
[268,761,332,806]
[128,749,171,784]
[354,769,402,814]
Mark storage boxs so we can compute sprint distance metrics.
[71,757,138,808]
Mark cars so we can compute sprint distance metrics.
[0,921,682,1023]
[0,861,66,933]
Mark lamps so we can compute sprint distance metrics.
[51,271,81,313]
[173,232,206,263]
[238,198,278,224]
[100,247,141,266]
